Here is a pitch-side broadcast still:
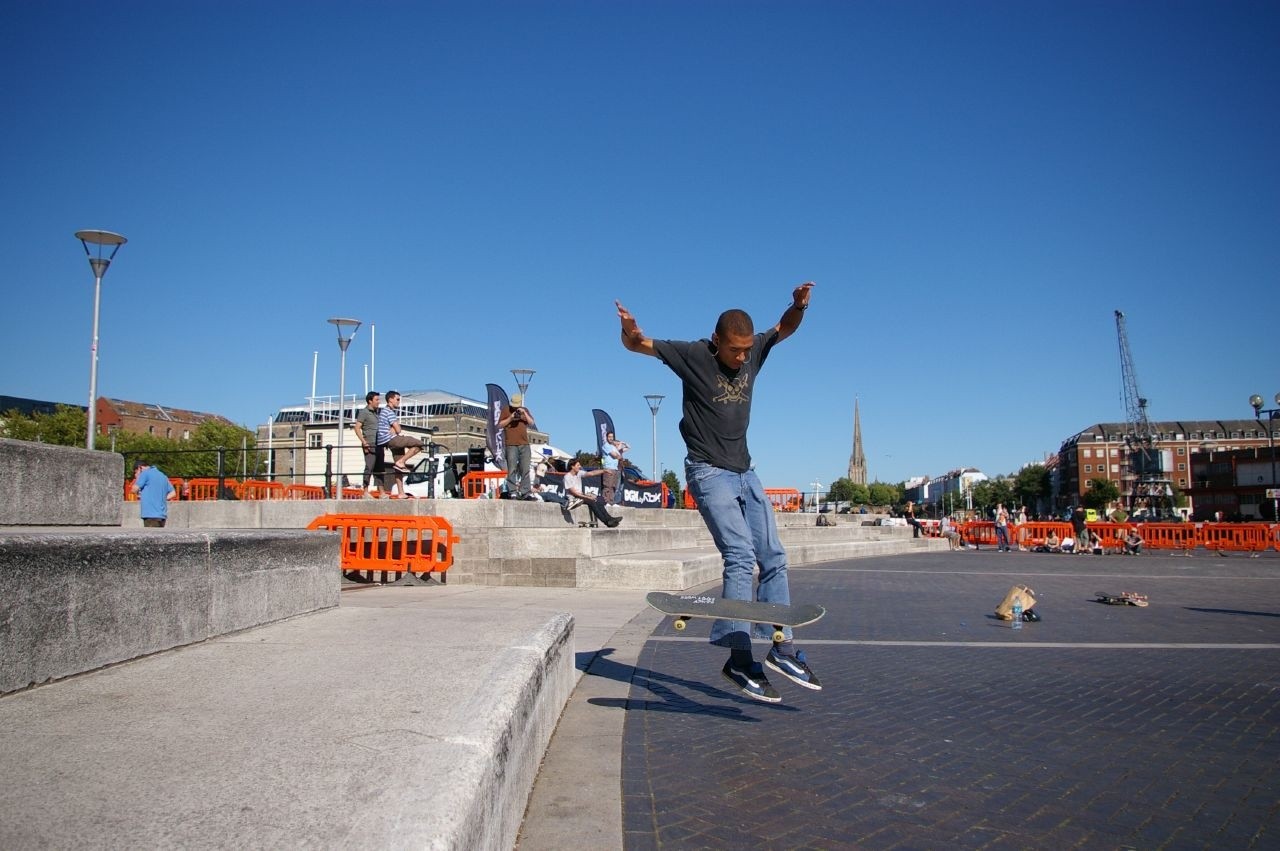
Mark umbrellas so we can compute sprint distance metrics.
[529,444,575,460]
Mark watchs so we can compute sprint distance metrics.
[794,304,808,312]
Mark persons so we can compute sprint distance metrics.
[129,459,177,528]
[940,499,1147,557]
[496,395,540,501]
[355,390,424,501]
[613,279,823,705]
[905,501,927,538]
[562,430,631,528]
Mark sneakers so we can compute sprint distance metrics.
[764,648,822,690]
[722,656,781,702]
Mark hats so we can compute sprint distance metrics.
[510,395,521,408]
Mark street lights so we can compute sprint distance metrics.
[644,395,665,483]
[660,462,664,476]
[325,318,363,499]
[75,229,128,449]
[1249,393,1280,523]
[511,369,536,407]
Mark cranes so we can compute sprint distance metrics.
[1113,309,1174,520]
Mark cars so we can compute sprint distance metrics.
[557,466,675,508]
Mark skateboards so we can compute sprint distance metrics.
[578,521,598,527]
[645,591,826,643]
[1095,591,1130,605]
[1121,591,1149,607]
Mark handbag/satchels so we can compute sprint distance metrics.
[997,584,1037,619]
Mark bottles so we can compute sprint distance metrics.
[1011,596,1022,629]
[490,481,496,498]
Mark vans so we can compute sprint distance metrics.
[388,451,537,498]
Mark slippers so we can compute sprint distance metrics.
[393,464,412,473]
[399,495,416,500]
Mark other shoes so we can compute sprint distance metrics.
[1122,591,1149,601]
[1125,596,1149,607]
[362,493,374,499]
[380,494,389,499]
[568,497,584,510]
[607,516,622,526]
